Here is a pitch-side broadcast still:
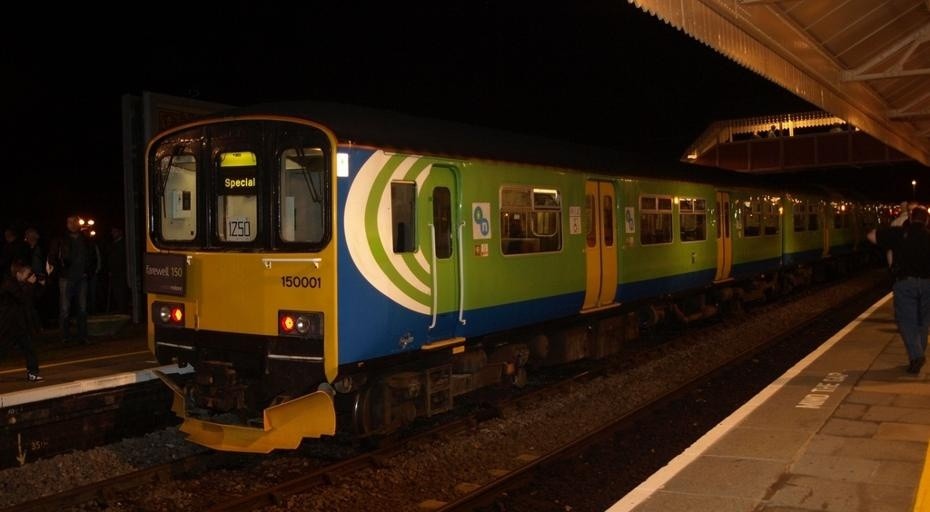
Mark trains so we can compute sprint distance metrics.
[142,116,860,454]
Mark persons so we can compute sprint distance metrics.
[768,125,776,138]
[867,201,930,375]
[1,207,145,385]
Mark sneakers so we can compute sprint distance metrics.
[906,358,925,374]
[24,369,45,383]
